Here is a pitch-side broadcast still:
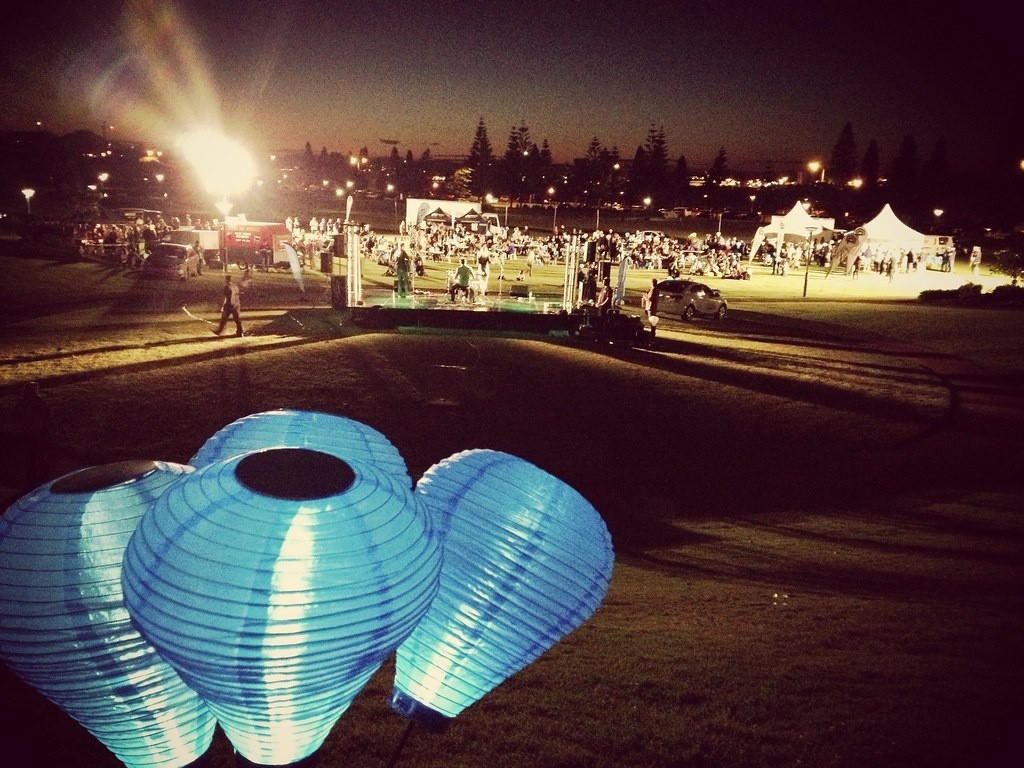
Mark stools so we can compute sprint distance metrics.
[454,286,470,303]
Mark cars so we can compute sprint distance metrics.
[144,243,200,281]
[643,279,728,323]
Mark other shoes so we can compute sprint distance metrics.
[236,333,243,337]
[211,329,219,335]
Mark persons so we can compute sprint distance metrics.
[74,212,983,337]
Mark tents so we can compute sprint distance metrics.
[425,207,488,236]
[762,200,926,260]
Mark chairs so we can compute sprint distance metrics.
[508,249,516,260]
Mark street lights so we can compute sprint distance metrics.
[351,157,360,172]
[22,189,36,218]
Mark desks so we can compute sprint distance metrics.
[496,249,507,260]
[427,251,444,263]
[444,271,486,302]
[535,254,550,265]
